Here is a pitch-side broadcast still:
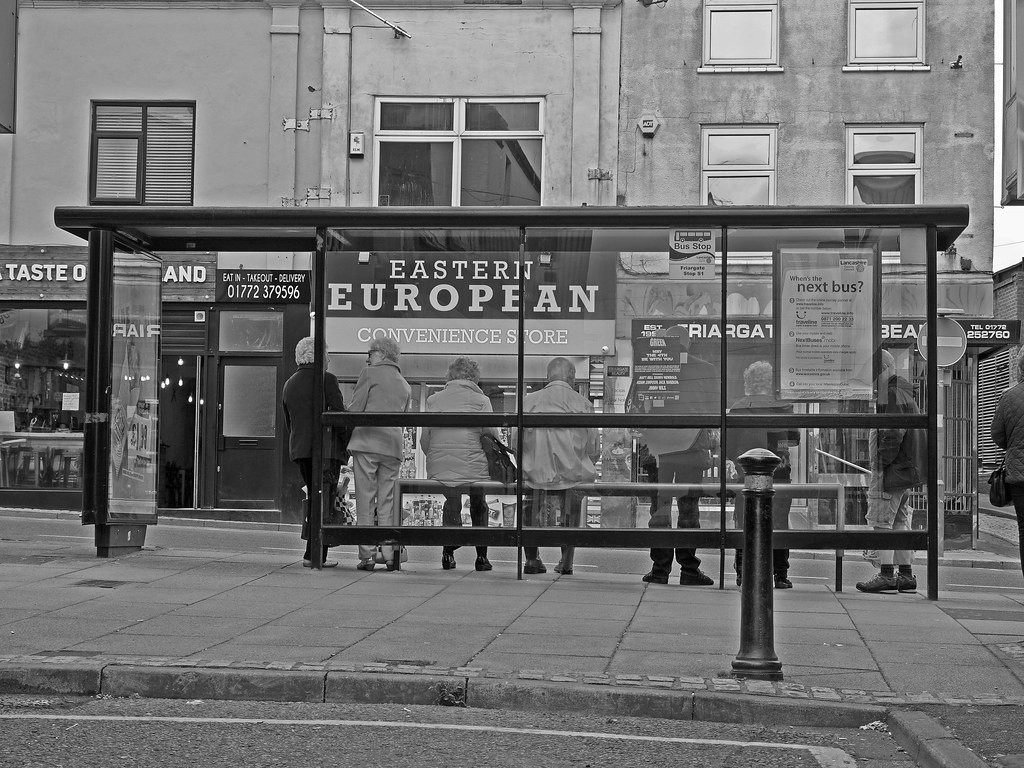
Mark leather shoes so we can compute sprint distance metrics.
[554,562,573,574]
[524,559,547,573]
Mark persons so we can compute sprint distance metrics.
[724,360,801,587]
[512,357,602,575]
[856,350,929,594]
[343,337,414,576]
[990,346,1023,576]
[420,356,506,571]
[280,335,346,566]
[624,326,722,586]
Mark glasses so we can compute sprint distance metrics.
[368,350,378,357]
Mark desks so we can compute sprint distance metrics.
[0,431,84,488]
[175,469,193,507]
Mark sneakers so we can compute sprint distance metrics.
[680,572,714,585]
[855,573,899,594]
[642,571,668,584]
[894,571,917,593]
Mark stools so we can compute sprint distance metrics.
[15,451,47,488]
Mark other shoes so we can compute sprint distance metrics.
[776,579,792,588]
[303,558,338,567]
[474,557,492,571]
[356,560,375,571]
[442,554,456,569]
[387,564,402,571]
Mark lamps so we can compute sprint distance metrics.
[59,353,71,371]
[15,370,20,378]
[59,371,84,381]
[11,351,24,370]
[123,355,204,405]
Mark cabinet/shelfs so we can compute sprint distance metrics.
[156,445,168,508]
[14,407,59,433]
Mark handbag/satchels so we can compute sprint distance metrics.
[481,433,517,484]
[987,456,1015,507]
[335,496,355,526]
[372,545,408,564]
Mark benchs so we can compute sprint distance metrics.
[393,478,845,592]
